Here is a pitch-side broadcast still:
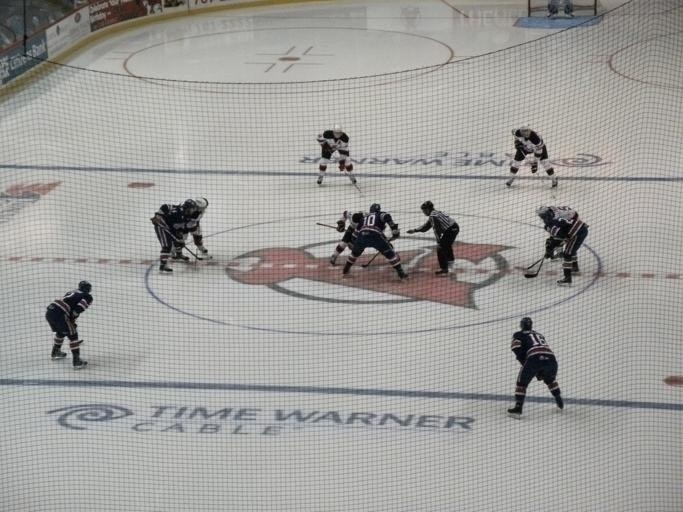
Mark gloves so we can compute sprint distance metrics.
[337,221,345,232]
[532,162,538,173]
[338,159,345,170]
[391,223,399,237]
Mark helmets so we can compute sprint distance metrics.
[334,125,343,137]
[519,124,531,137]
[536,203,549,215]
[79,281,90,292]
[420,201,433,215]
[369,204,380,213]
[182,198,206,214]
[520,317,533,330]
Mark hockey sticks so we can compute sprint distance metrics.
[521,150,556,200]
[155,221,213,261]
[526,257,546,278]
[327,146,361,194]
[361,238,392,267]
[516,258,542,270]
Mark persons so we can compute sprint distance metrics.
[149,199,198,272]
[316,123,359,185]
[169,197,209,257]
[405,200,460,275]
[538,208,590,284]
[504,124,559,188]
[507,316,565,415]
[342,202,408,279]
[45,279,95,367]
[544,0,576,18]
[0,0,102,53]
[536,205,578,260]
[330,209,368,263]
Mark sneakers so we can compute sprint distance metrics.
[506,178,514,187]
[436,258,455,274]
[175,254,189,260]
[330,256,348,274]
[159,266,172,271]
[508,407,521,414]
[400,272,407,278]
[558,402,564,409]
[317,175,323,184]
[51,350,66,357]
[352,176,356,183]
[552,176,558,187]
[72,361,87,366]
[556,265,579,283]
[198,247,207,252]
